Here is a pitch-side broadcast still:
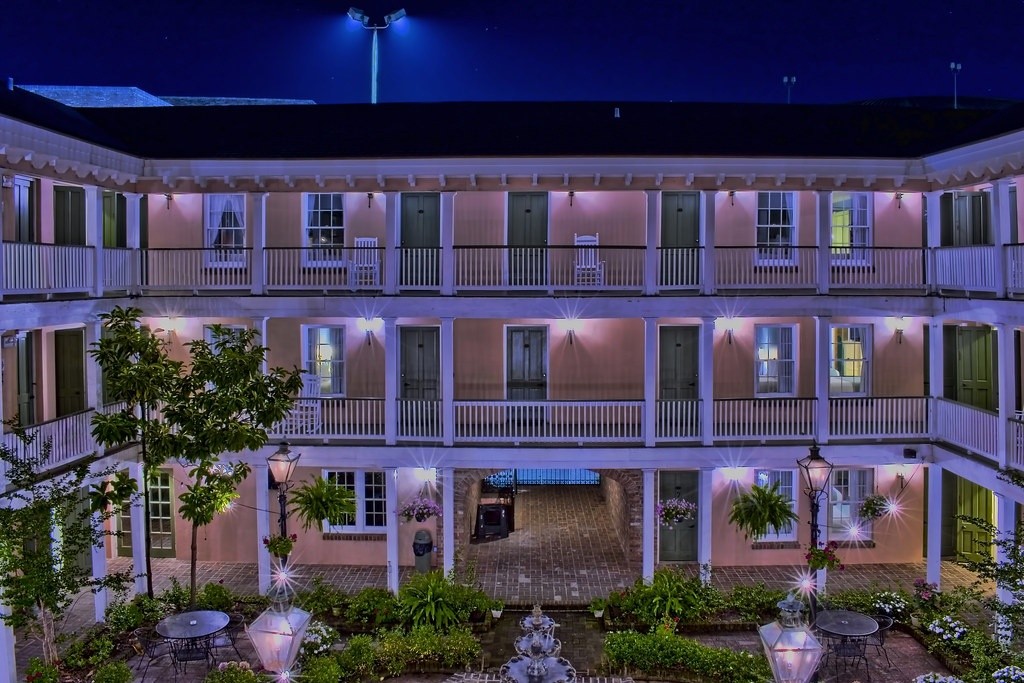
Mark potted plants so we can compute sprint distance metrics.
[327,591,344,618]
[730,479,800,543]
[286,472,358,532]
[589,596,611,618]
[490,600,506,618]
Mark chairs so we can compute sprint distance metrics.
[808,608,894,682]
[134,602,244,683]
[293,375,325,435]
[350,237,383,287]
[571,232,607,287]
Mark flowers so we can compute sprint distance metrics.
[913,579,941,619]
[853,494,895,524]
[394,499,446,527]
[262,532,298,555]
[656,496,693,526]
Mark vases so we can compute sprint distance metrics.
[673,515,684,524]
[910,614,926,627]
[413,514,424,522]
[276,552,286,558]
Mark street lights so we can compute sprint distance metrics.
[267,441,303,568]
[795,438,833,617]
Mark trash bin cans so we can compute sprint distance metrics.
[412,530,433,574]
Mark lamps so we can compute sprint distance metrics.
[161,316,177,349]
[364,318,378,347]
[565,317,579,346]
[897,463,907,489]
[420,466,431,494]
[893,315,912,344]
[724,316,737,344]
[161,188,906,212]
